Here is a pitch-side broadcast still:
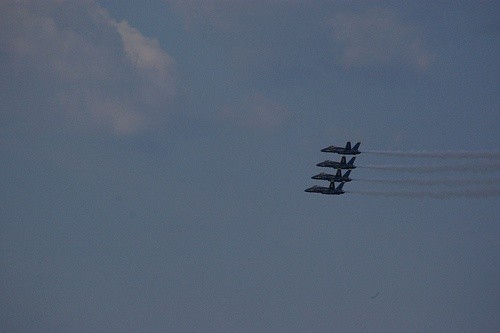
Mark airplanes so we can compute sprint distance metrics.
[311,169,352,183]
[320,141,361,155]
[304,182,345,195]
[315,156,357,169]
